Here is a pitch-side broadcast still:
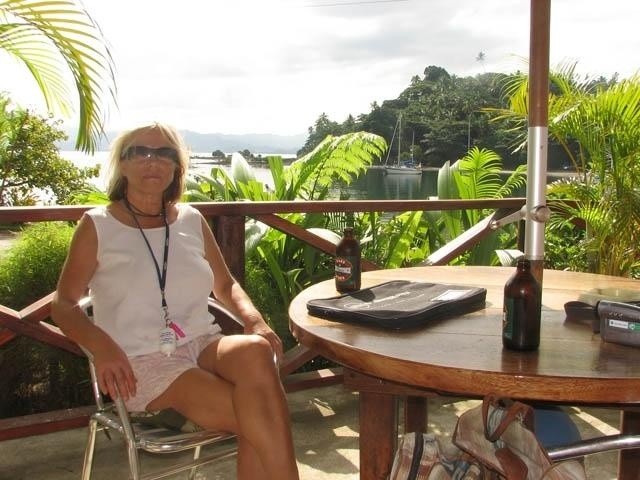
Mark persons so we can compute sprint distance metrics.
[47,118,305,479]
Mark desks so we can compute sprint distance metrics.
[284,260,640,480]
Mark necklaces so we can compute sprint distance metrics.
[128,200,162,218]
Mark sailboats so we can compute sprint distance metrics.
[385,111,422,174]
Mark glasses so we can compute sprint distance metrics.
[118,144,178,164]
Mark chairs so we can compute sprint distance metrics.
[72,293,279,480]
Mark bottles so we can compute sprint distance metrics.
[334,228,362,293]
[501,258,544,354]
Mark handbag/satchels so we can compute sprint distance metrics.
[384,394,587,480]
[305,280,488,336]
